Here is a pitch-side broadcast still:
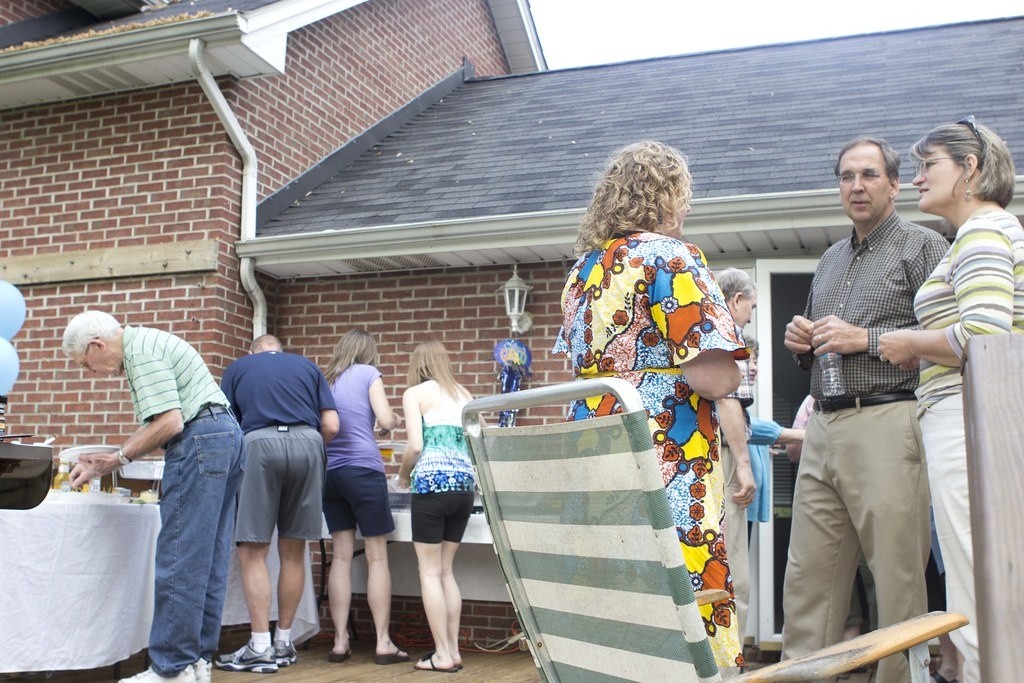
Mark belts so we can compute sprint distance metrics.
[812,392,916,411]
[195,407,232,418]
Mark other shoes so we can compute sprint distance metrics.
[931,672,958,683]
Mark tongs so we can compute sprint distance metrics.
[379,425,397,436]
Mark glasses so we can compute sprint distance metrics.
[915,156,963,174]
[837,170,881,182]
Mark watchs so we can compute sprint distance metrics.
[118,449,132,466]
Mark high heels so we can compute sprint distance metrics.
[328,650,351,662]
[375,648,410,664]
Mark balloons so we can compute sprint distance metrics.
[0,279,27,397]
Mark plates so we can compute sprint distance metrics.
[377,443,407,454]
[60,447,120,461]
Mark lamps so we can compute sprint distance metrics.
[499,262,535,335]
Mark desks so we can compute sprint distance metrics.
[0,495,507,677]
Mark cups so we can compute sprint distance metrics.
[141,489,159,504]
[113,486,131,503]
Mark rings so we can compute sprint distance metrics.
[819,334,822,342]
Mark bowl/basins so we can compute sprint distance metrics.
[388,493,412,508]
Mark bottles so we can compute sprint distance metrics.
[817,339,847,397]
[50,454,113,493]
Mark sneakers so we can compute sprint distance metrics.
[273,640,298,666]
[215,640,279,672]
[194,658,212,683]
[118,665,196,683]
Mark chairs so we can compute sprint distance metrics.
[461,377,968,683]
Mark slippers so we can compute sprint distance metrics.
[415,656,458,672]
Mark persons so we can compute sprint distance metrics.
[220,334,340,674]
[715,266,816,677]
[399,341,488,671]
[781,392,876,673]
[556,137,742,680]
[320,329,411,664]
[61,310,248,683]
[875,121,1024,683]
[780,135,951,682]
